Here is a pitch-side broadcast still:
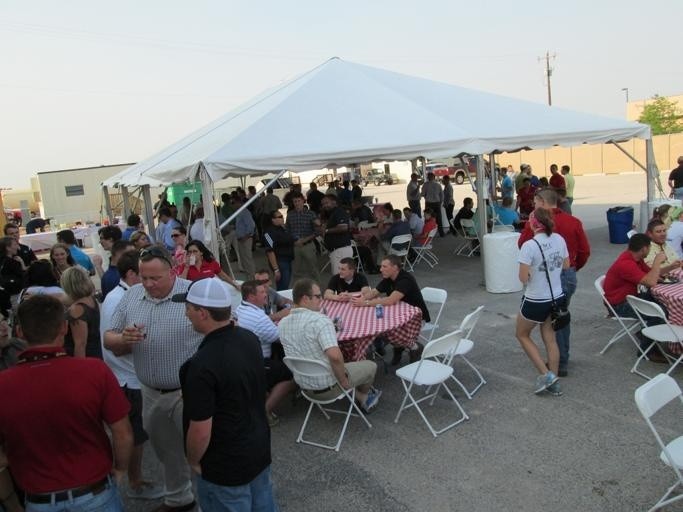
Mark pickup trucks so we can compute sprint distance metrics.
[361,168,393,185]
[432,156,500,184]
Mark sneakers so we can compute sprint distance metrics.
[533,370,563,397]
[362,386,383,414]
[128,480,163,498]
[265,408,278,425]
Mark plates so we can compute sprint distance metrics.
[657,274,680,284]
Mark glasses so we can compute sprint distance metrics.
[170,233,184,239]
[306,294,324,299]
[258,278,272,284]
[532,189,544,204]
[269,213,283,219]
[138,247,168,261]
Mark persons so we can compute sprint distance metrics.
[452,164,590,397]
[282,179,410,282]
[668,155,682,200]
[277,254,431,413]
[602,205,683,366]
[403,172,454,263]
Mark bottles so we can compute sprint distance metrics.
[190,253,196,266]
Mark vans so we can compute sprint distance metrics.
[416,162,447,185]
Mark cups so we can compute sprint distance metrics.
[352,294,361,305]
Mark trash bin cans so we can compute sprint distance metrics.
[606,206,634,244]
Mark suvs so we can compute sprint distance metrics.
[336,171,350,185]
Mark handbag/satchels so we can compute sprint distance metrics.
[531,237,572,331]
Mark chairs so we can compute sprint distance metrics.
[357,219,370,231]
[284,358,373,451]
[411,225,438,271]
[415,225,440,265]
[432,305,492,398]
[627,226,640,242]
[490,225,514,233]
[594,273,651,360]
[362,223,377,229]
[625,291,683,384]
[632,374,683,512]
[334,239,370,274]
[395,329,467,435]
[277,289,297,304]
[385,235,415,276]
[449,218,475,257]
[313,234,335,276]
[458,219,481,256]
[401,286,448,349]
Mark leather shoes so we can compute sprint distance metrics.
[410,343,424,365]
[390,346,404,366]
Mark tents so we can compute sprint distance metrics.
[99,55,655,263]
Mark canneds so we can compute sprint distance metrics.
[334,316,343,331]
[376,303,383,318]
[284,303,290,309]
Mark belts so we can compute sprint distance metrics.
[298,382,340,397]
[22,474,114,504]
[153,386,181,396]
[301,239,312,247]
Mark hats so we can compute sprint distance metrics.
[172,275,232,309]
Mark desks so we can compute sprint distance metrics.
[650,275,683,354]
[275,294,424,366]
[352,228,389,247]
[19,228,71,252]
